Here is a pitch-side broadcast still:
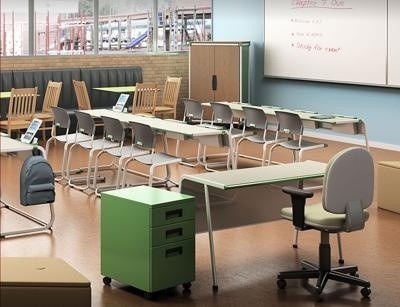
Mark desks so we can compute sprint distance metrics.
[179,161,344,294]
[74,102,368,189]
[0,134,55,238]
[0,92,40,119]
[92,85,160,112]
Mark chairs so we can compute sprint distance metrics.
[45,97,327,196]
[278,146,374,299]
[31,80,63,146]
[132,83,157,145]
[141,76,182,120]
[72,78,104,126]
[0,86,37,140]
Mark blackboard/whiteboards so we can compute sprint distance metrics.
[262,0,400,88]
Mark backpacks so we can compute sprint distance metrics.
[20,150,55,206]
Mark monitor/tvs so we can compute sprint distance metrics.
[20,118,43,144]
[113,93,131,112]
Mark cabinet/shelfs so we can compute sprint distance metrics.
[378,161,400,215]
[188,41,250,123]
[0,256,91,307]
[100,185,195,300]
